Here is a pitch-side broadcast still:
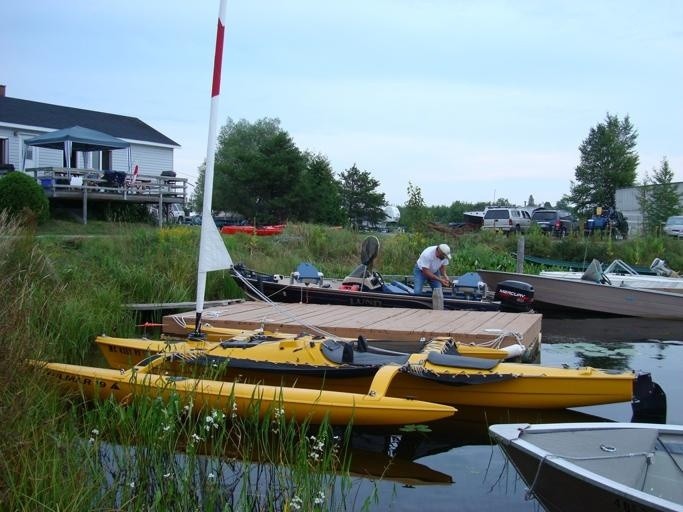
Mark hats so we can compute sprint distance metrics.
[438,243,451,259]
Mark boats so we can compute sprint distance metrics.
[479,416,683,512]
[21,357,458,429]
[56,406,458,490]
[226,262,536,323]
[220,223,290,235]
[96,300,653,410]
[474,257,683,323]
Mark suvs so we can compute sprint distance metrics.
[478,204,627,241]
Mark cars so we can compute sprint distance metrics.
[167,204,185,224]
[660,215,682,239]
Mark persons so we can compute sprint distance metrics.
[414,244,452,296]
